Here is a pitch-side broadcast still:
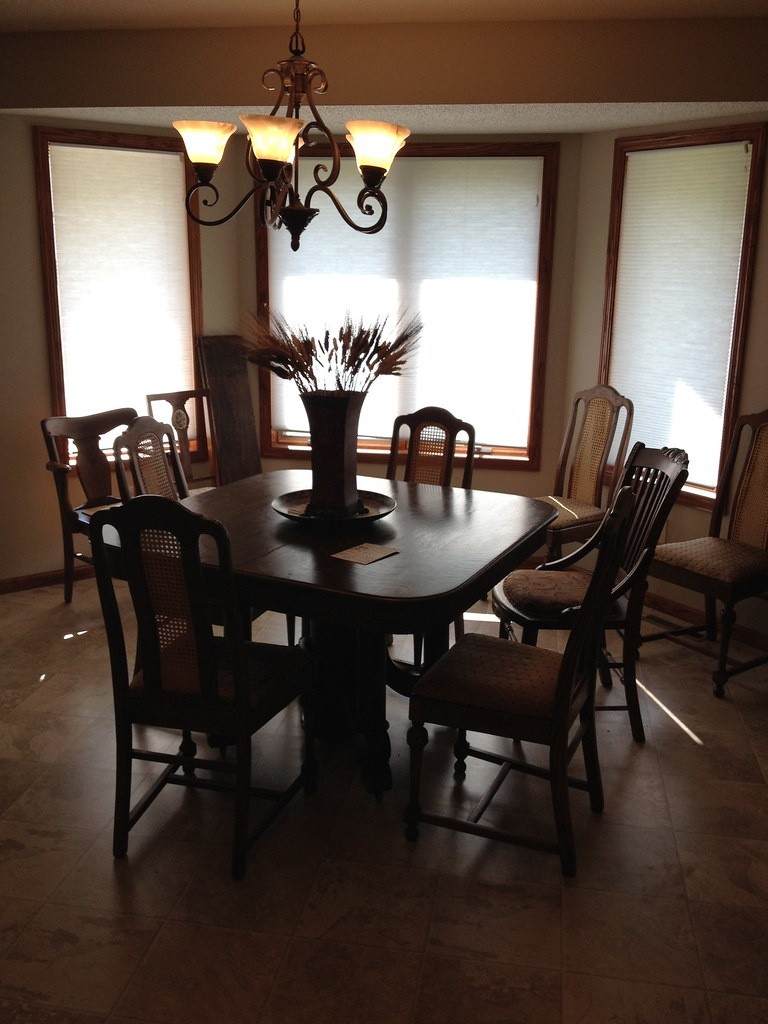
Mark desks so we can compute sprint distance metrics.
[138,468,558,801]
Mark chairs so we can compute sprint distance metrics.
[491,442,689,745]
[480,386,634,602]
[401,486,637,883]
[42,408,145,605]
[632,410,768,696]
[89,494,318,880]
[378,407,475,670]
[115,331,297,646]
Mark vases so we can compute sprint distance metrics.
[299,391,369,514]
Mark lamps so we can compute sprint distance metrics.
[170,0,411,251]
